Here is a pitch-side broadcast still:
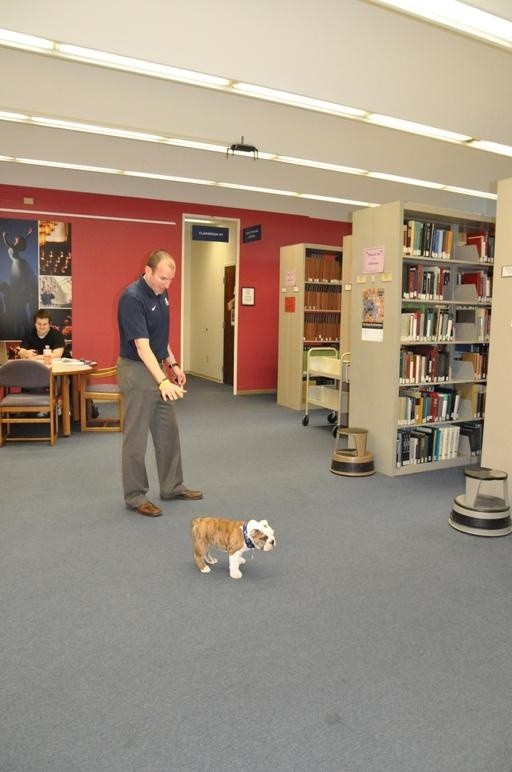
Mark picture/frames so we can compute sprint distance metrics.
[241,286,256,307]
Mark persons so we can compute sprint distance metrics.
[115,248,204,517]
[17,310,99,424]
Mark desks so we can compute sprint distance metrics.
[23,357,95,438]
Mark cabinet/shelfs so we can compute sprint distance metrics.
[337,235,352,426]
[277,242,343,412]
[477,178,511,504]
[352,198,495,480]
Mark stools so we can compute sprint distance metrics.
[447,465,511,537]
[328,427,376,477]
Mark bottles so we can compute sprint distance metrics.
[43,345,52,366]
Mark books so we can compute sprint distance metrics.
[395,217,495,469]
[302,252,341,402]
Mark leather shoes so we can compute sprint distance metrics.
[127,500,160,517]
[160,489,203,499]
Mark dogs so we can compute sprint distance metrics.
[191,516,277,579]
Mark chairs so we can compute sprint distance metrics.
[80,366,127,433]
[0,358,62,447]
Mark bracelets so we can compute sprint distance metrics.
[158,378,171,390]
[168,362,179,369]
